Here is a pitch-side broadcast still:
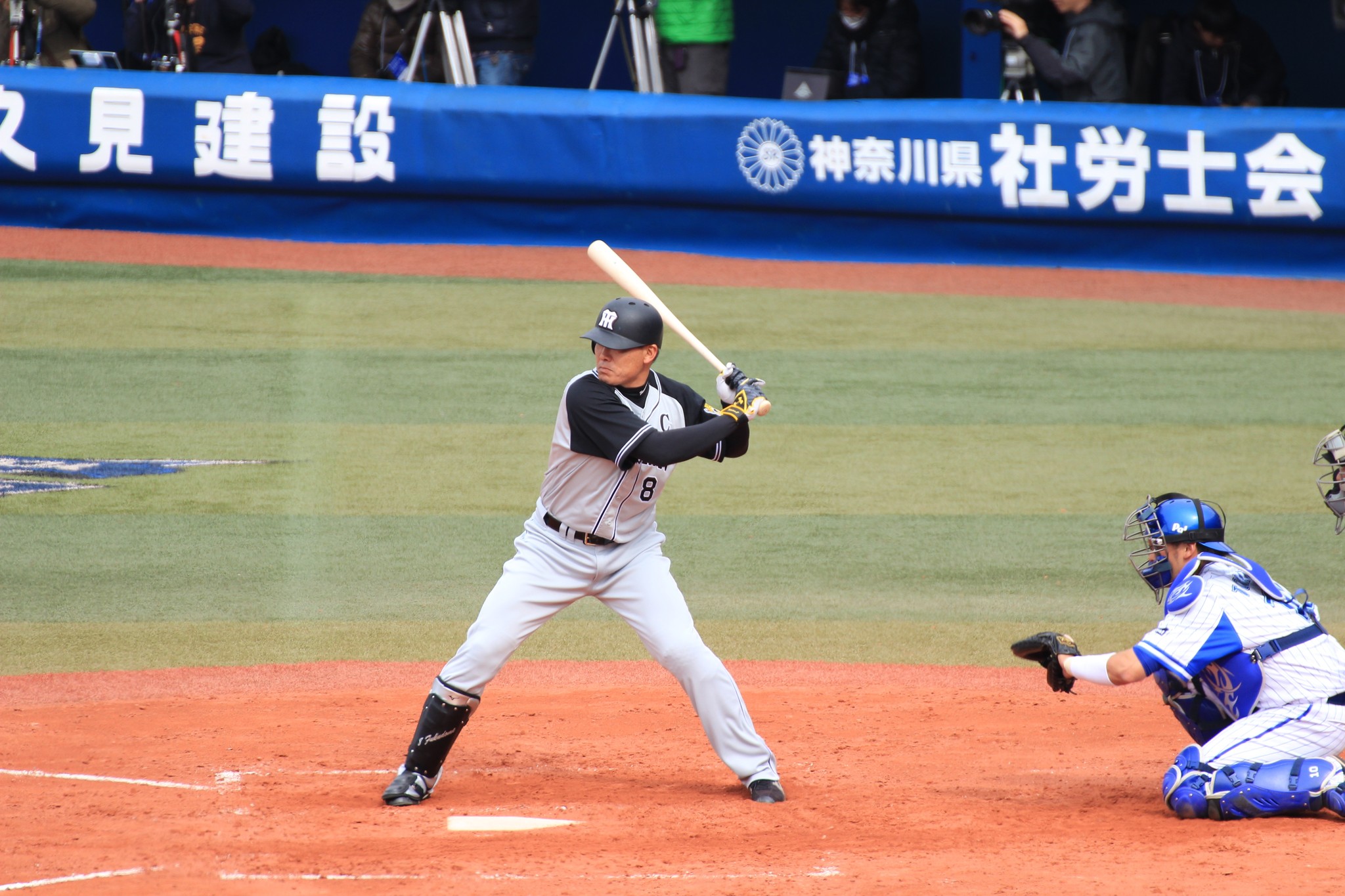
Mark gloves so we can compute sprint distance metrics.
[715,361,749,409]
[721,377,766,426]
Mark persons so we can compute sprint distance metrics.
[998,0,1282,109]
[379,297,785,807]
[1011,492,1345,821]
[123,0,257,74]
[0,0,97,67]
[1313,425,1345,536]
[651,0,735,97]
[348,0,540,86]
[814,0,923,100]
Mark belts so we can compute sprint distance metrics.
[543,512,613,547]
[1326,691,1345,706]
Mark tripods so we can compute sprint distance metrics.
[999,40,1040,101]
[586,0,666,93]
[401,0,476,86]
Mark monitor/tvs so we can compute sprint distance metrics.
[69,48,122,69]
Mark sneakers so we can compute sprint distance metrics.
[747,780,786,805]
[1323,754,1345,817]
[381,764,438,806]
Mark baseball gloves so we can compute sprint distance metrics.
[1011,631,1083,692]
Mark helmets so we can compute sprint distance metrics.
[1321,424,1345,519]
[1136,493,1238,590]
[579,296,663,350]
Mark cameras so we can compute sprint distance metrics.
[967,6,1004,34]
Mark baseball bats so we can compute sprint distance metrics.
[587,240,771,417]
